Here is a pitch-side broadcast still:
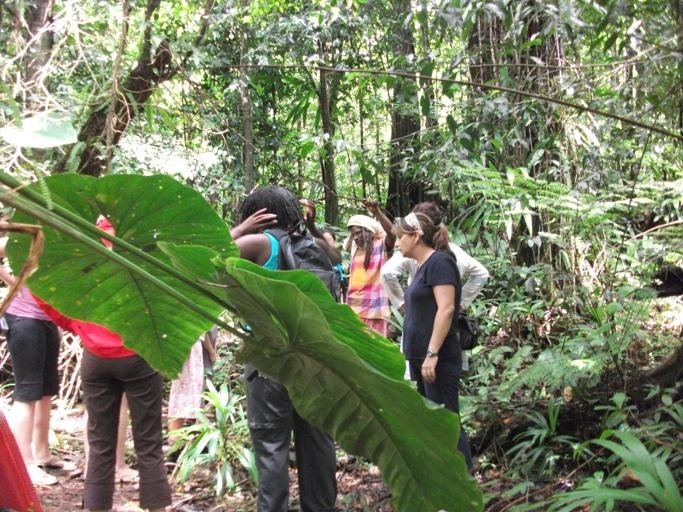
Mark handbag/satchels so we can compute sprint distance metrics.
[459,316,480,351]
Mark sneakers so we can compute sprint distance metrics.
[28,466,58,485]
[39,455,65,469]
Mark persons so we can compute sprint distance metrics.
[344,196,489,478]
[228,185,341,512]
[0,213,218,512]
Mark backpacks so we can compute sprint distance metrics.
[265,227,341,302]
